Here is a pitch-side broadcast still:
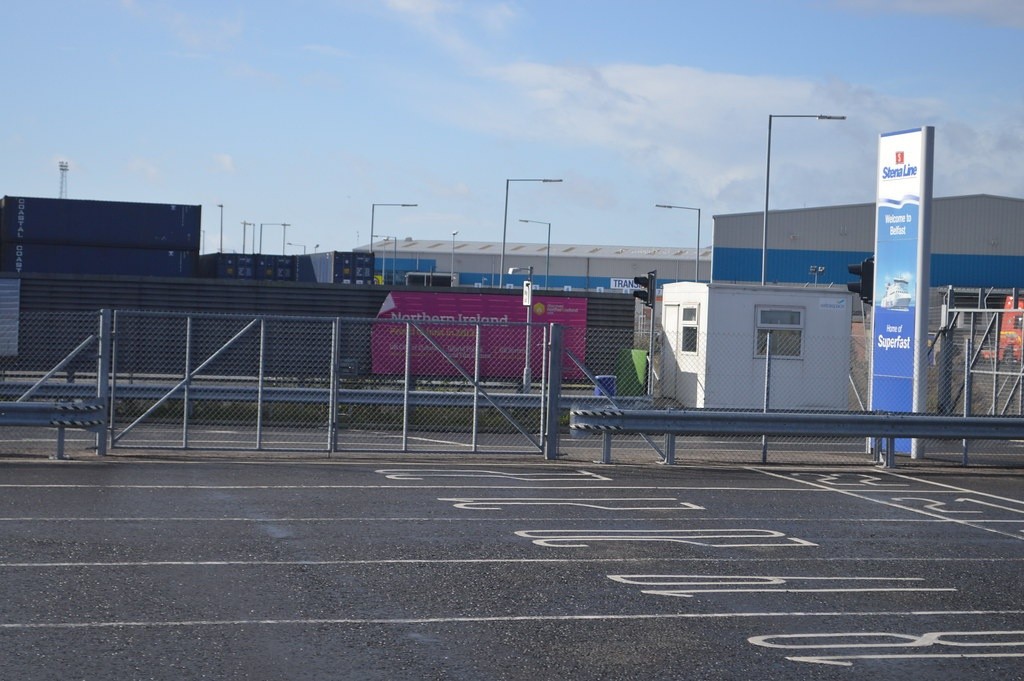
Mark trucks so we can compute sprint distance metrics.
[928,295,1024,366]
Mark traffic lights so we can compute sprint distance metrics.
[848,260,874,307]
[632,271,657,308]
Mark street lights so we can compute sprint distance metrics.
[241,220,252,253]
[314,243,319,254]
[370,203,419,252]
[287,243,307,256]
[372,235,398,286]
[759,113,846,289]
[498,176,562,289]
[216,203,224,253]
[654,203,701,284]
[281,223,291,255]
[508,265,533,393]
[518,219,552,290]
[451,230,460,287]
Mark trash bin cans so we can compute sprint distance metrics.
[595,375,617,396]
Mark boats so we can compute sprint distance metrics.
[880,278,911,309]
